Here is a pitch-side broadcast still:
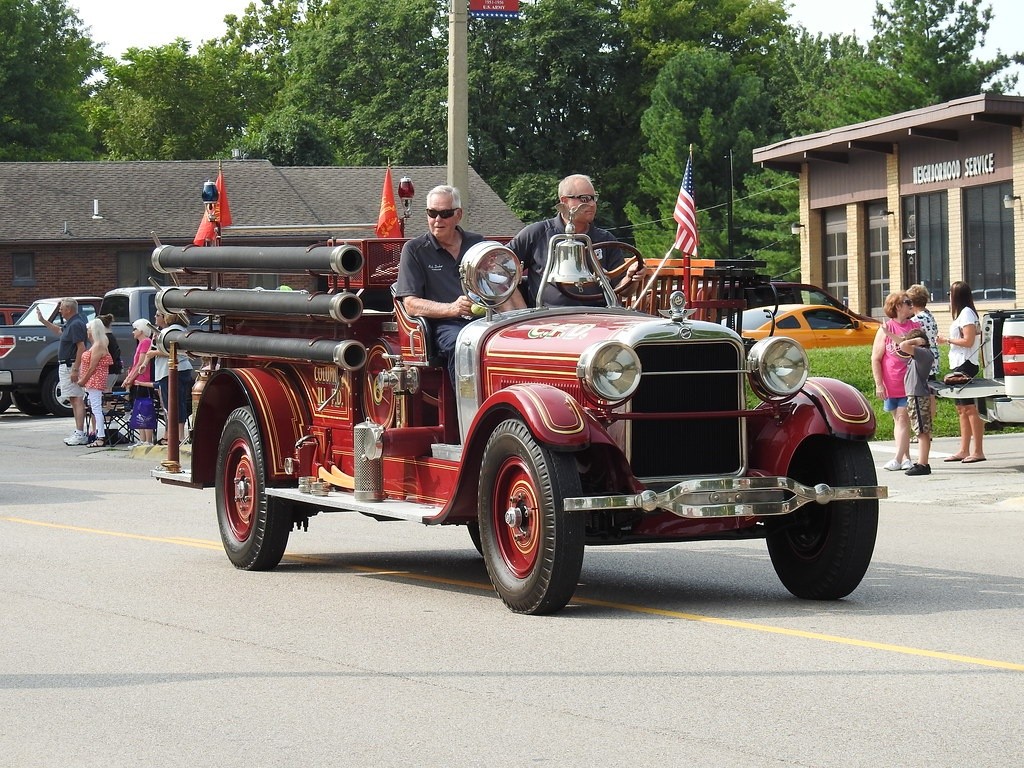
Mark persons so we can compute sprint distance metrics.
[138,308,196,446]
[36,297,92,446]
[121,320,157,451]
[906,284,940,443]
[77,316,114,448]
[936,281,987,463]
[881,326,934,476]
[872,289,924,471]
[95,313,122,421]
[394,184,516,400]
[503,174,648,311]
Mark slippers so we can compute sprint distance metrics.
[961,455,987,463]
[943,455,965,462]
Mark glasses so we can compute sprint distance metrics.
[903,299,912,306]
[426,208,460,219]
[946,291,951,295]
[133,328,137,331]
[564,194,599,202]
[155,314,164,317]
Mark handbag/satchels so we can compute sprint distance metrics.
[129,386,157,429]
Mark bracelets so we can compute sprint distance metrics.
[73,368,78,371]
[946,338,950,345]
[142,363,147,367]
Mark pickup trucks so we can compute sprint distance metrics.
[144,232,889,617]
[0,286,221,418]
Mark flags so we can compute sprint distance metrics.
[672,155,699,258]
[375,168,402,239]
[193,174,232,247]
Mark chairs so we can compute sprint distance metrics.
[84,383,191,446]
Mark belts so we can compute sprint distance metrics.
[59,360,75,365]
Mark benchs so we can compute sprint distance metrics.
[388,275,529,444]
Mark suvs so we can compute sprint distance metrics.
[645,279,883,324]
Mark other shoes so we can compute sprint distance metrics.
[128,441,154,451]
[883,459,901,471]
[900,459,911,469]
[88,432,98,443]
[905,463,931,475]
[86,439,106,447]
[66,433,88,446]
[155,438,169,446]
[63,431,81,443]
[179,439,188,445]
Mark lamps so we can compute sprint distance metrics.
[791,222,805,234]
[1003,194,1021,209]
[880,209,894,221]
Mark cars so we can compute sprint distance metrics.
[720,304,883,349]
